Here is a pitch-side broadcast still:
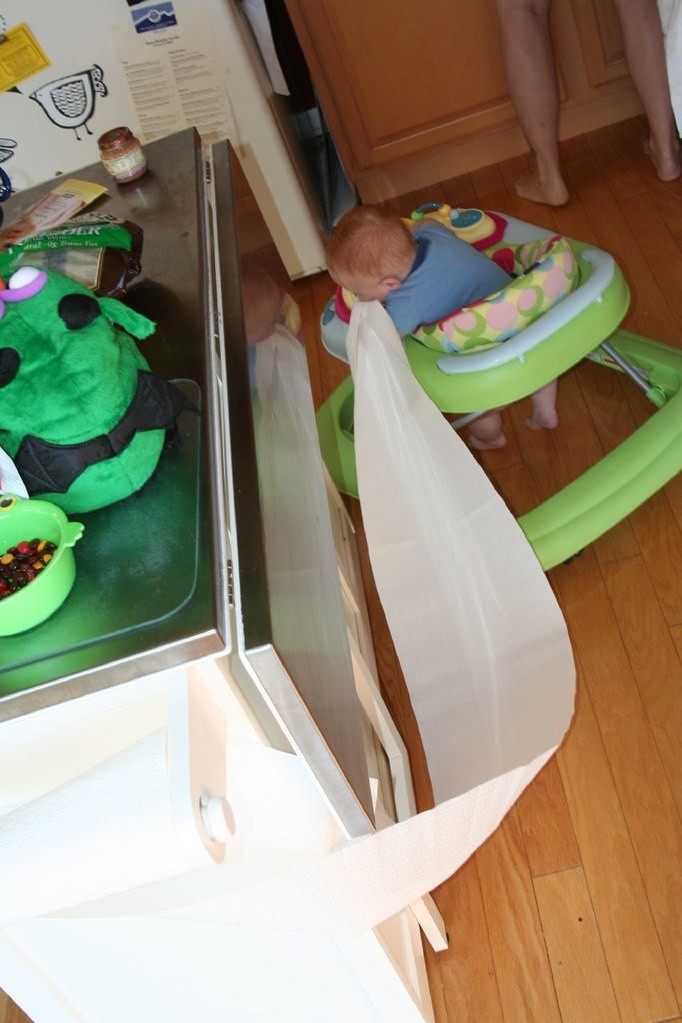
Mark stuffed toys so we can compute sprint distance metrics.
[1,263,175,513]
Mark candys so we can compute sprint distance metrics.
[0,537,56,601]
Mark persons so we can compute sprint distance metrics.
[493,0,682,204]
[326,204,557,449]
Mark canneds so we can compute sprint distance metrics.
[97,126,148,184]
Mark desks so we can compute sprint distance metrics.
[0,125,448,1023]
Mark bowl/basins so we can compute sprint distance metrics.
[0,493,85,638]
